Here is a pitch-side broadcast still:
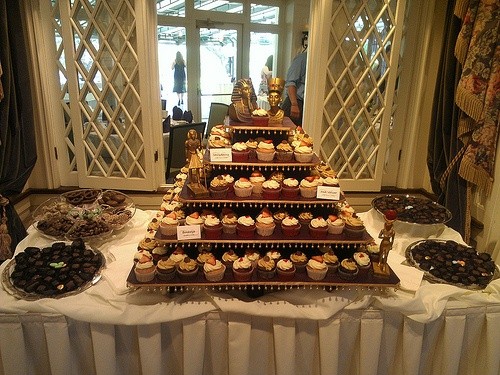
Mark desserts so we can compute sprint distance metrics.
[207,125,313,163]
[250,107,269,126]
[133,167,379,281]
[197,148,339,198]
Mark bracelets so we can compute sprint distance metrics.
[291,105,296,106]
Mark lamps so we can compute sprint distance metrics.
[302,25,309,47]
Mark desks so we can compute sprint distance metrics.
[0,209,500,375]
[86,131,170,159]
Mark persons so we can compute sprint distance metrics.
[229,77,257,115]
[285,54,306,126]
[185,130,200,162]
[267,78,285,124]
[257,55,273,96]
[172,51,186,105]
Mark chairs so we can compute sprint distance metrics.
[165,121,207,179]
[205,102,229,139]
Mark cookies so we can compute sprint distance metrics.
[45,202,131,227]
[11,237,102,296]
[376,195,449,224]
[36,216,109,238]
[98,190,126,207]
[411,240,496,285]
